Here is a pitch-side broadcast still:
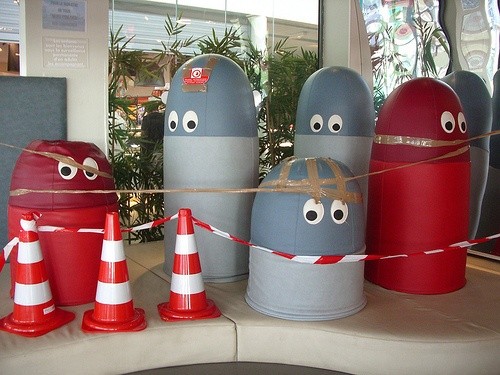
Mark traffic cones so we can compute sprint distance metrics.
[81,211,148,334]
[0,212,76,338]
[157,209,222,321]
